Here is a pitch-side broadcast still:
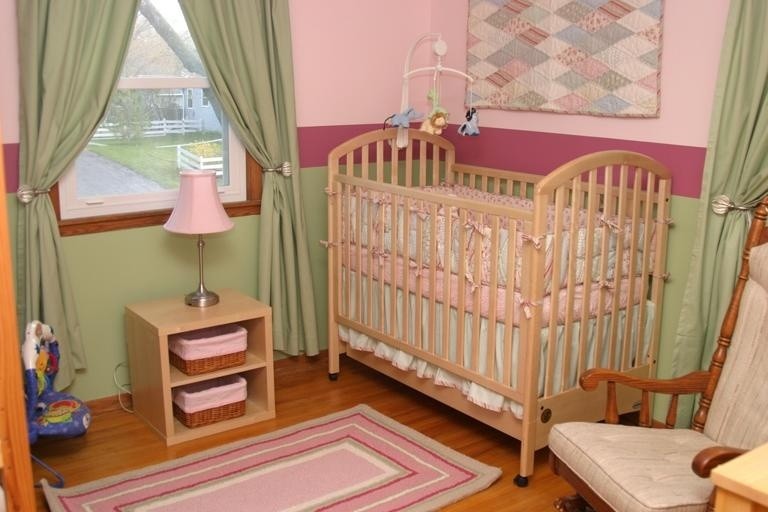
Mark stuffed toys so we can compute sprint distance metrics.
[396,32,481,149]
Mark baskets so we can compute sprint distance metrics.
[169,324,245,375]
[173,378,246,429]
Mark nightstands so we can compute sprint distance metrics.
[124,286,277,447]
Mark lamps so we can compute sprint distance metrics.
[162,169,236,307]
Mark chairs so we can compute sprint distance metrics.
[544,195,768,512]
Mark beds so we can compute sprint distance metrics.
[320,126,673,488]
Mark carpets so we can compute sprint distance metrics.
[39,401,504,512]
